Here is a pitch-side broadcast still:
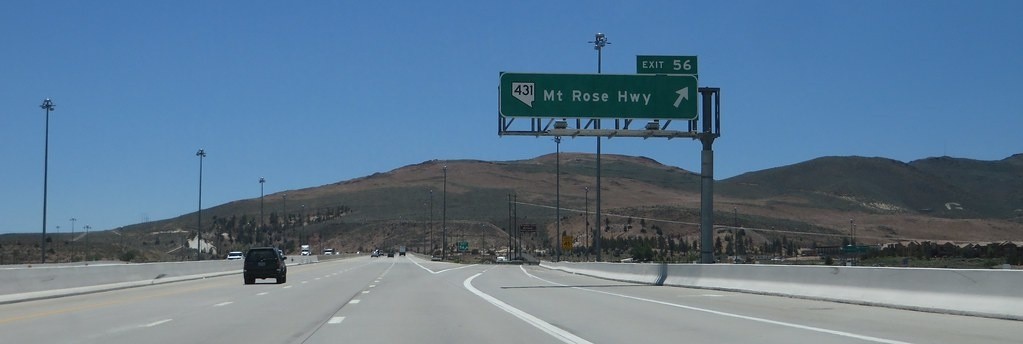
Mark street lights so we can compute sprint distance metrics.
[429,190,433,257]
[196,148,206,261]
[584,186,590,248]
[40,97,56,261]
[84,224,90,260]
[589,32,611,260]
[258,177,266,247]
[69,217,77,261]
[554,135,561,262]
[442,165,448,259]
[282,193,288,247]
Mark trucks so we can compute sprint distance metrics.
[300,244,312,256]
[399,246,407,257]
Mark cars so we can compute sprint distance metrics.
[227,251,243,260]
[325,248,333,255]
[371,248,396,258]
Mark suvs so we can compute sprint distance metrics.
[244,246,288,284]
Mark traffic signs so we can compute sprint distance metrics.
[497,70,700,121]
